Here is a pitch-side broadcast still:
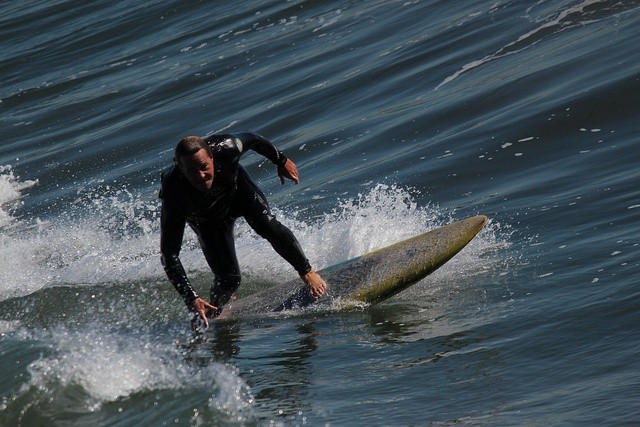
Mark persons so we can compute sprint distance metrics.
[160,131,327,334]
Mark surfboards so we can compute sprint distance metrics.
[189,214,487,321]
